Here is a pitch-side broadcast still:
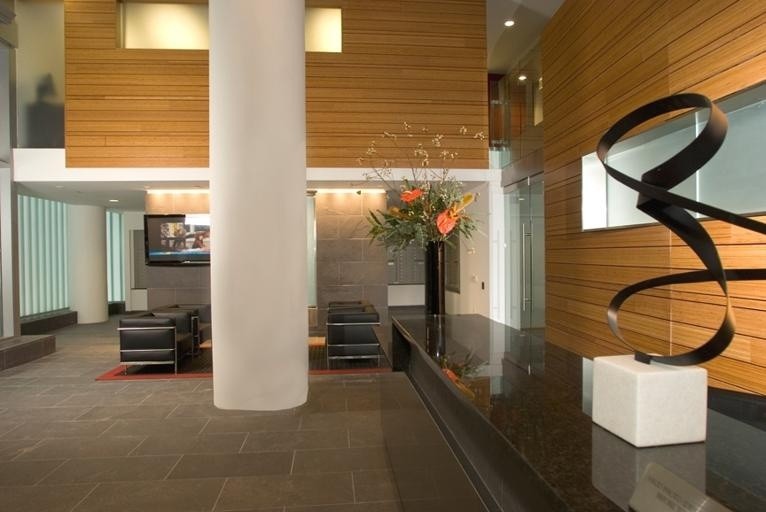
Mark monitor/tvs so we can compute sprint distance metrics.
[144,214,210,267]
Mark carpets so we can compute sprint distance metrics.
[93,344,390,381]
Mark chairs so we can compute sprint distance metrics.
[118,302,211,376]
[324,298,382,369]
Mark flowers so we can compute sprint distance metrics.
[357,120,486,256]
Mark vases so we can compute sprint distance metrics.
[425,238,445,316]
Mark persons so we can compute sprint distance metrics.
[191,233,205,249]
[174,224,187,249]
